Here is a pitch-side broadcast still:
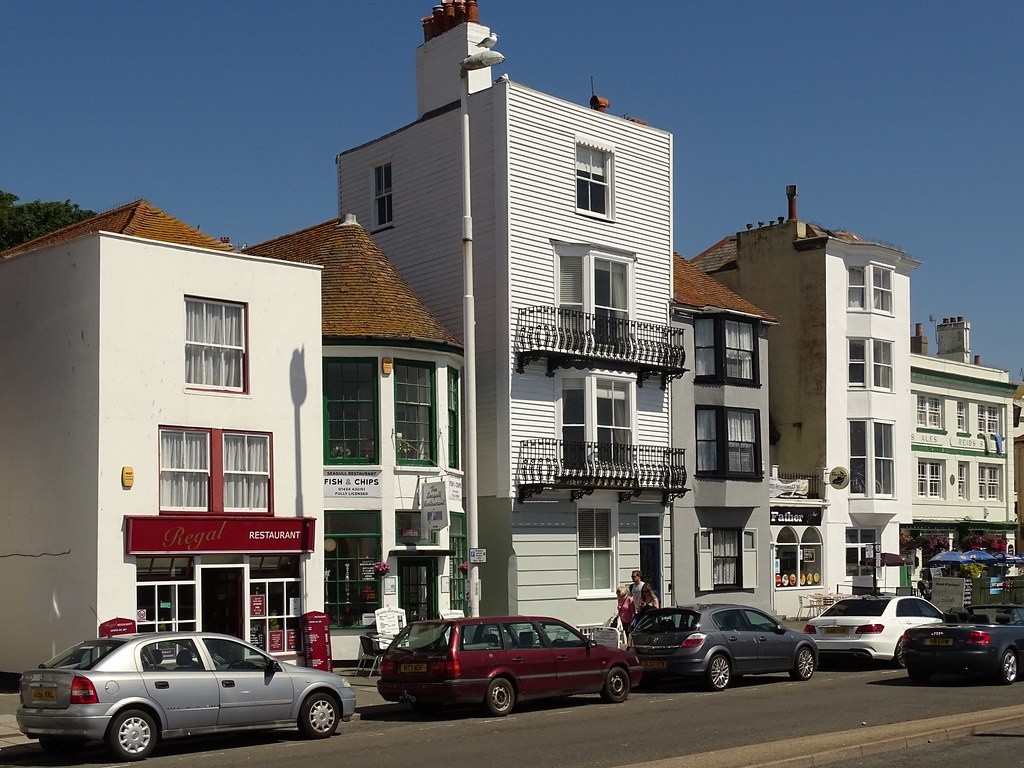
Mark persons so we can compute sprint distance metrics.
[637,583,659,612]
[616,586,636,640]
[629,570,646,615]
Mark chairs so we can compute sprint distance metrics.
[796,592,850,621]
[718,614,741,631]
[918,581,932,600]
[354,630,395,678]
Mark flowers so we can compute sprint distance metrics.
[373,561,390,572]
[458,561,468,571]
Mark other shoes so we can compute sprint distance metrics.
[626,646,630,651]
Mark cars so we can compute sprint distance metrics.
[803,595,944,668]
[15,631,357,761]
[625,603,820,690]
[377,616,640,717]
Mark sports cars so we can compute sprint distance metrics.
[900,603,1024,685]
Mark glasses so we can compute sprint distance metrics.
[631,576,635,578]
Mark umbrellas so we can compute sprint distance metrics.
[962,551,997,576]
[927,551,976,578]
[858,551,914,593]
[991,553,1024,577]
